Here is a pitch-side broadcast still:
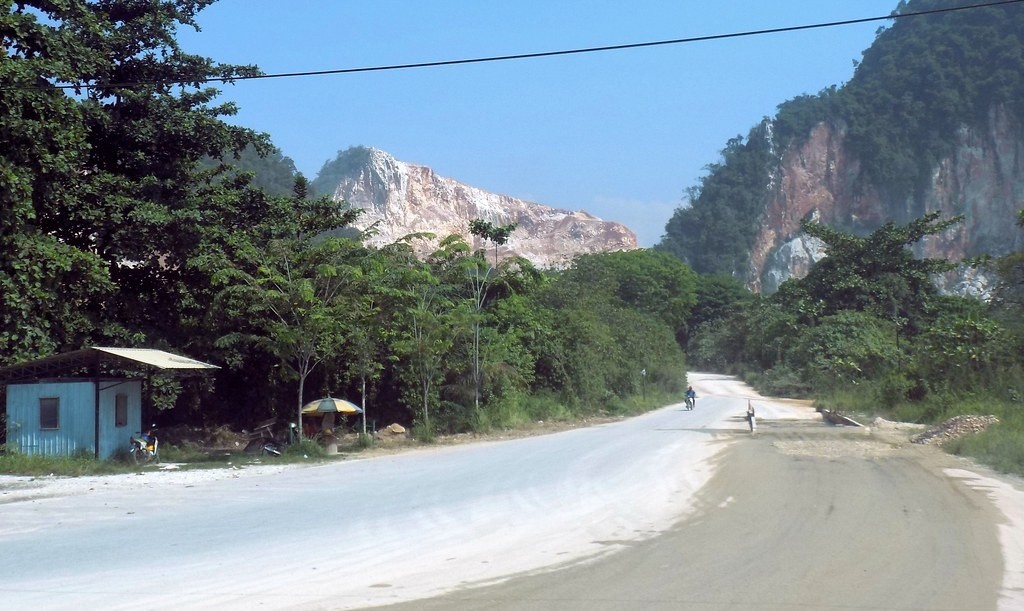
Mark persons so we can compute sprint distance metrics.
[684,386,695,406]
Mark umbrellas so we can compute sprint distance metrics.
[300,397,363,415]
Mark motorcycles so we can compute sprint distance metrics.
[129,423,161,465]
[684,395,695,411]
